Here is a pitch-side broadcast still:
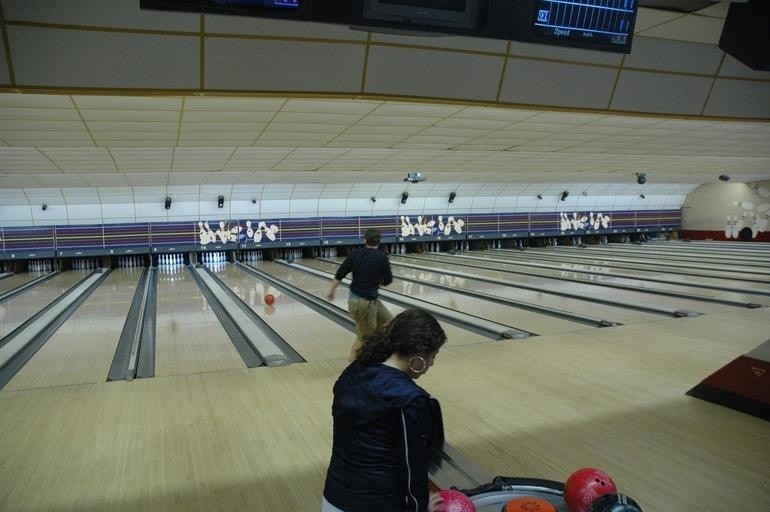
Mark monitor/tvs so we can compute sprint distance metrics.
[349,0,478,37]
[140,0,310,21]
[524,0,642,54]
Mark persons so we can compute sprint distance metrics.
[320,306,449,511]
[326,228,394,364]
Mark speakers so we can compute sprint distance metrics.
[561,191,568,201]
[718,0,770,71]
[165,197,171,209]
[218,195,224,208]
[449,192,456,202]
[401,192,408,203]
